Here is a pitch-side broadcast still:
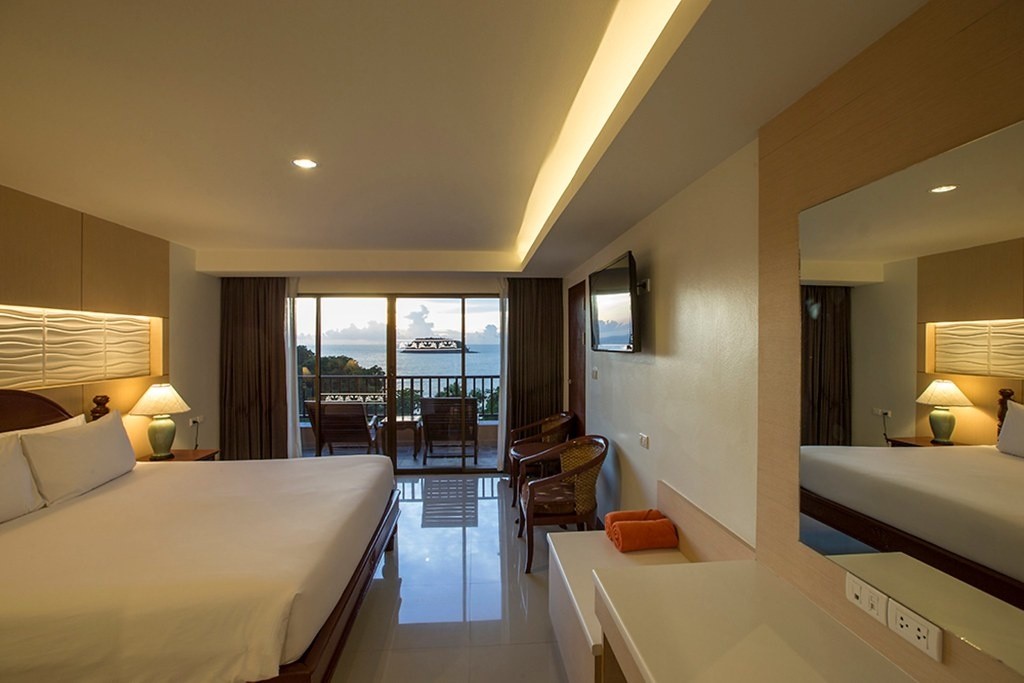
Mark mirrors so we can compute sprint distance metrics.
[755,0,1024,675]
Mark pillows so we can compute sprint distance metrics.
[20,409,137,507]
[995,398,1024,457]
[0,413,87,438]
[0,433,46,524]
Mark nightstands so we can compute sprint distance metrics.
[889,436,971,447]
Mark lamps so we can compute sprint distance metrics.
[126,382,192,461]
[916,378,977,445]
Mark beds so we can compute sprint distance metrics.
[0,387,403,683]
[797,387,1024,611]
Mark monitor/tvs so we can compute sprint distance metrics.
[588,251,642,354]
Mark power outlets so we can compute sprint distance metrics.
[189,417,196,426]
[639,433,649,450]
[882,409,892,418]
[845,571,888,627]
[872,407,882,416]
[888,598,944,665]
[196,415,204,424]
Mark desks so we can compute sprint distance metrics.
[827,551,1024,676]
[510,442,561,479]
[379,414,422,460]
[594,559,919,683]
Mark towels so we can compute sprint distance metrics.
[612,518,679,552]
[605,508,662,539]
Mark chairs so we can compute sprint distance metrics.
[509,410,577,508]
[420,476,479,528]
[304,399,379,455]
[420,396,479,466]
[517,434,610,577]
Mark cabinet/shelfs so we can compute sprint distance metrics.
[136,449,221,461]
[544,530,694,683]
